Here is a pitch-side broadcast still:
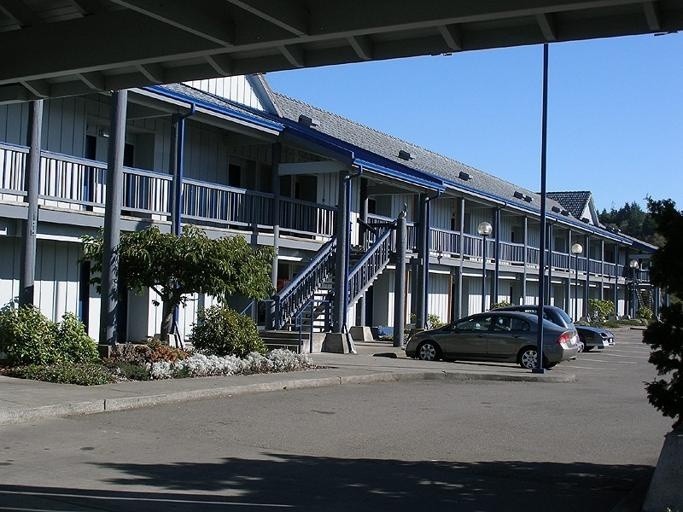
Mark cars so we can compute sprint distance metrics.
[525,312,618,354]
[406,311,580,370]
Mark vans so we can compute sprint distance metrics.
[459,303,586,357]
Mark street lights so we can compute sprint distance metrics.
[570,244,583,326]
[476,221,493,313]
[629,259,640,320]
